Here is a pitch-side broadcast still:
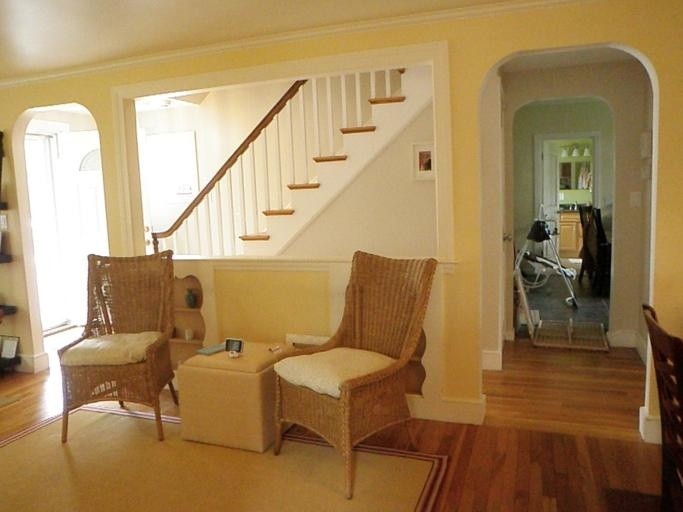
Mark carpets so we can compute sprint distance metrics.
[0,406,450,510]
[533,321,610,352]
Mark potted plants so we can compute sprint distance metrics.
[560,143,591,157]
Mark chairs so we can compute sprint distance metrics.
[642,303,683,512]
[578,206,611,293]
[273,247,438,501]
[58,248,178,447]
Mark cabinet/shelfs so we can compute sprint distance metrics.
[559,156,592,205]
[559,211,584,259]
[167,306,206,349]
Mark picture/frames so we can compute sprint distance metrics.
[410,140,435,182]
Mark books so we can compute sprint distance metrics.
[195,343,228,357]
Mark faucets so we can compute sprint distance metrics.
[569,202,573,210]
[574,200,577,211]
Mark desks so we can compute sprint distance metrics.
[178,338,286,456]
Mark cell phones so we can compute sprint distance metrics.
[225,339,243,353]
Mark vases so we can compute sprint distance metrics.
[183,286,196,309]
